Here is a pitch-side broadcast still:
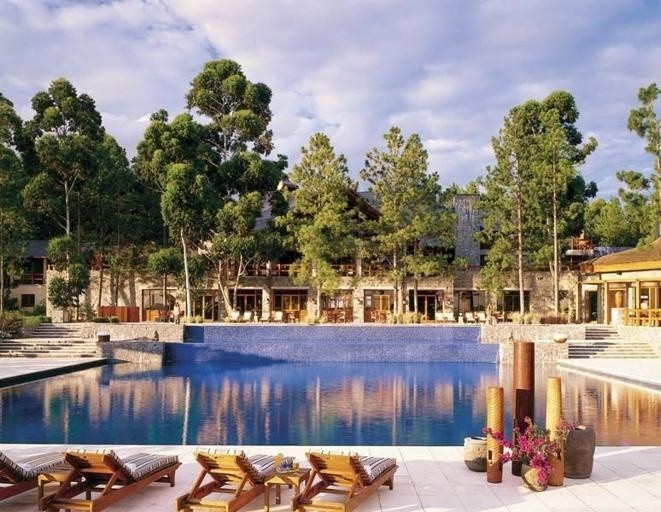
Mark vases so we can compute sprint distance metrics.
[519,461,550,491]
[276,465,293,476]
[463,436,489,472]
[564,423,595,480]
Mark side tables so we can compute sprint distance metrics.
[264,468,312,512]
[37,462,82,502]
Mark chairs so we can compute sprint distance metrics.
[291,451,397,512]
[156,306,184,323]
[0,449,75,502]
[37,448,182,512]
[225,306,519,323]
[174,448,294,512]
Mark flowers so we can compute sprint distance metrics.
[274,452,299,472]
[483,417,578,486]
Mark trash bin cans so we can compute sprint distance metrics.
[63,310,69,323]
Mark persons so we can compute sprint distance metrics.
[172,302,180,324]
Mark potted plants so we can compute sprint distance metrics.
[48,262,90,323]
[611,290,625,327]
